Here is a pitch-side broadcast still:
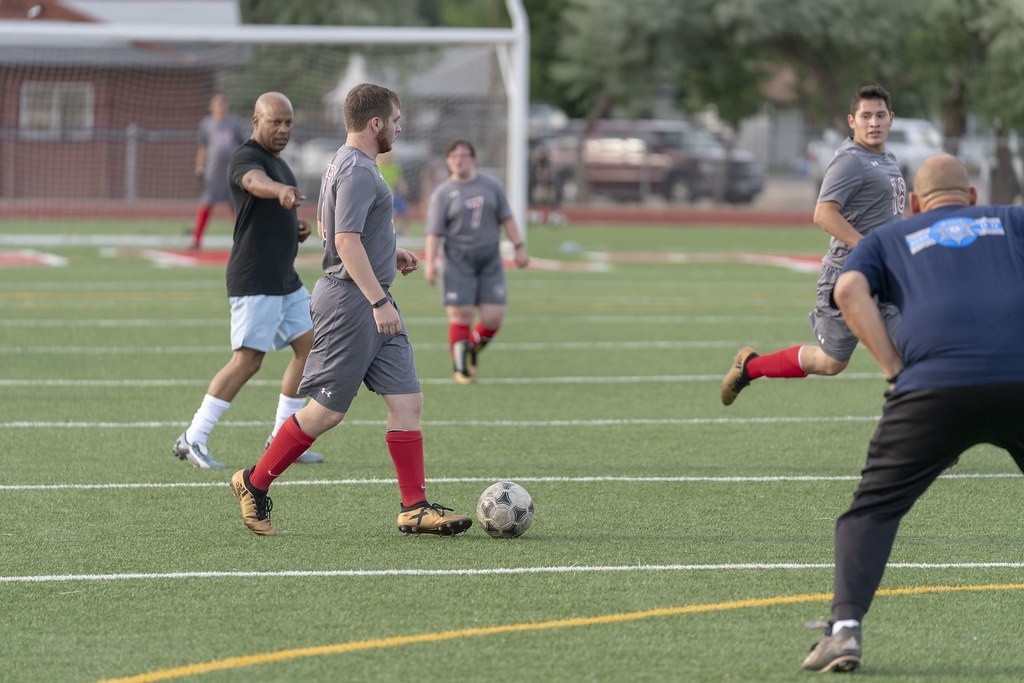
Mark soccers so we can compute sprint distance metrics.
[475,480,535,539]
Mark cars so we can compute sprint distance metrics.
[813,116,945,207]
[567,118,764,208]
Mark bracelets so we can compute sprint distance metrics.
[230,83,476,537]
[883,364,907,385]
[513,239,526,251]
[370,296,388,310]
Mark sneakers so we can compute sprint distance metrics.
[265,436,324,463]
[397,500,473,536]
[229,465,276,536]
[720,347,756,405]
[173,431,224,468]
[801,619,861,672]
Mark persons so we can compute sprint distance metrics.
[172,91,325,469]
[183,90,243,252]
[799,152,1024,683]
[423,139,529,386]
[720,86,907,407]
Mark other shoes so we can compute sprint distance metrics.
[454,370,474,385]
[463,348,478,369]
